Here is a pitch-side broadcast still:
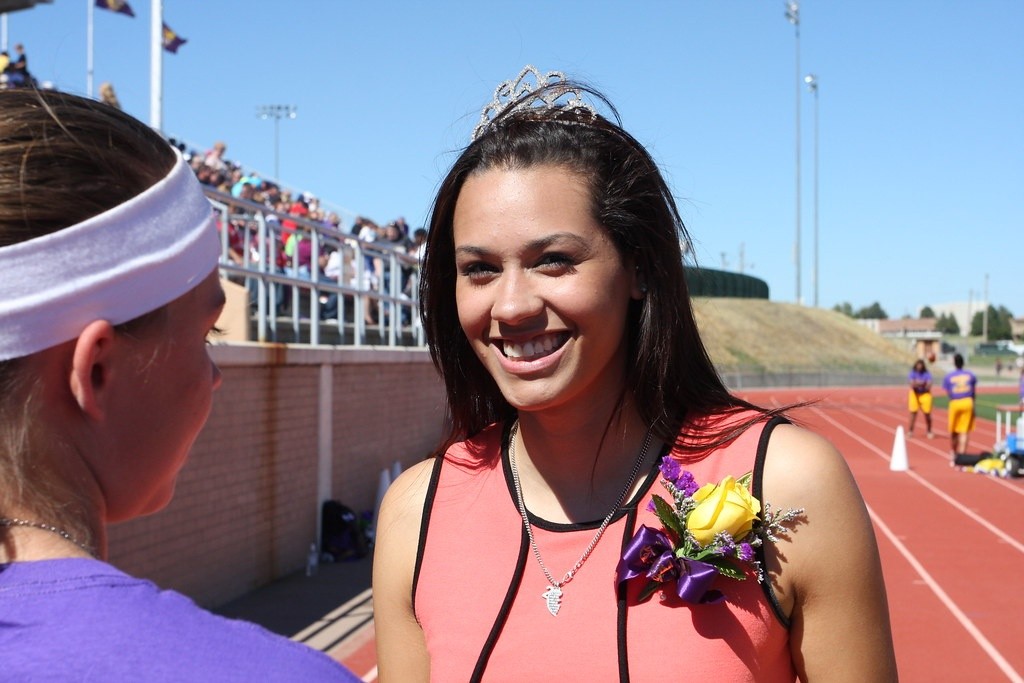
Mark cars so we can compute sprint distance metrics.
[974,343,1019,358]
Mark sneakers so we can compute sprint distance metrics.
[927,432,933,439]
[326,318,338,324]
[949,460,955,466]
[908,431,913,436]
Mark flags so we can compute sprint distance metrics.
[161,23,185,53]
[94,0,134,17]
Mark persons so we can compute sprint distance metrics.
[372,64,899,683]
[941,354,977,467]
[906,360,935,440]
[0,90,365,683]
[100,82,121,109]
[169,135,431,347]
[0,44,30,89]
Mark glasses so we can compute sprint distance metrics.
[332,218,338,227]
[323,254,328,264]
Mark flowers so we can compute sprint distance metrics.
[619,455,809,606]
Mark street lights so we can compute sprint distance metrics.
[805,75,821,311]
[259,103,298,182]
[783,0,803,306]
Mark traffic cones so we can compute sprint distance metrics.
[888,424,911,472]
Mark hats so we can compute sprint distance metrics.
[290,192,312,203]
[386,221,399,229]
[358,226,377,243]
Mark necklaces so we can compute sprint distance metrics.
[510,417,654,617]
[0,518,100,557]
